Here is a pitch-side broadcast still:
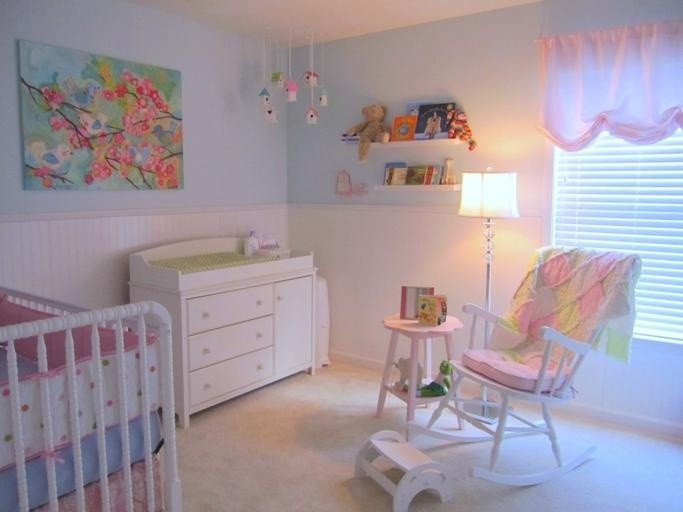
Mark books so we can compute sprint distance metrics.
[389,99,455,141]
[382,162,444,185]
[416,294,448,327]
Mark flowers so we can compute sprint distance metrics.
[20,71,184,190]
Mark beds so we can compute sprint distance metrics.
[0,285,184,512]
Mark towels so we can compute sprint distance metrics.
[487,245,642,374]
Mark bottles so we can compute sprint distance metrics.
[244,230,260,259]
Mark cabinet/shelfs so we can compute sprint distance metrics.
[353,138,468,193]
[127,236,320,430]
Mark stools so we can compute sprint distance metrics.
[375,311,464,442]
[352,429,458,512]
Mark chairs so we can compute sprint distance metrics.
[406,245,640,486]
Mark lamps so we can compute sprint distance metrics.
[457,172,520,419]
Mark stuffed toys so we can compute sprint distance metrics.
[447,109,477,150]
[415,359,451,398]
[394,357,424,392]
[346,104,389,160]
